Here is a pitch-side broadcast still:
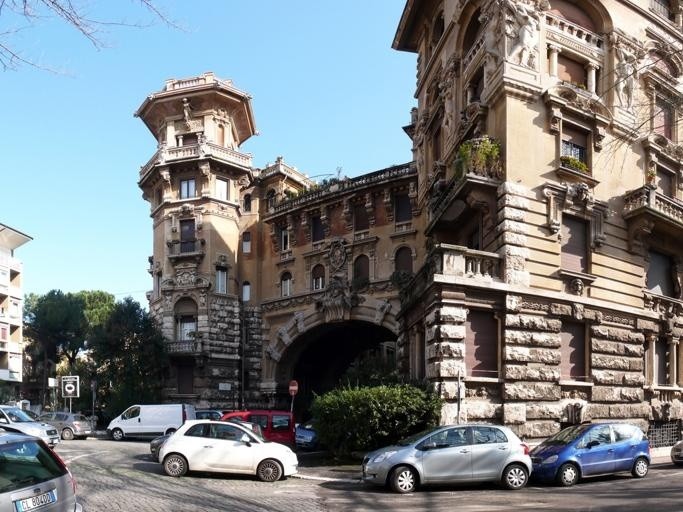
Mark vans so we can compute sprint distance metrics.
[219,410,296,451]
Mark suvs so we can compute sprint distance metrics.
[0,425,81,512]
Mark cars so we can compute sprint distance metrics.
[150,433,170,462]
[294,418,318,450]
[670,438,682,464]
[528,420,651,487]
[9,409,38,423]
[33,412,91,440]
[194,410,223,421]
[258,418,298,430]
[0,404,59,450]
[157,419,299,482]
[359,423,533,493]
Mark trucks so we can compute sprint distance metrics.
[106,404,196,440]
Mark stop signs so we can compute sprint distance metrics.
[288,380,298,396]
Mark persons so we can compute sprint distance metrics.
[438,67,454,137]
[613,43,641,116]
[477,4,504,76]
[408,127,427,178]
[507,0,541,70]
[180,97,195,126]
